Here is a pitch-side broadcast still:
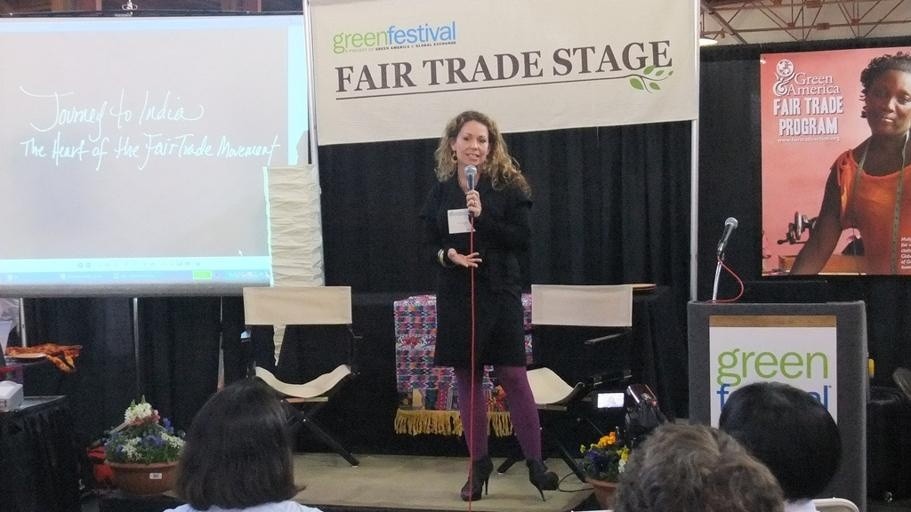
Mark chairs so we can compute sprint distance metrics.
[238,285,359,469]
[497,284,659,484]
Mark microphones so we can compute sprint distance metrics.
[464,164,477,216]
[715,216,739,261]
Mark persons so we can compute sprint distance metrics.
[614,419,782,510]
[720,383,839,511]
[416,111,559,503]
[162,376,320,511]
[789,53,909,274]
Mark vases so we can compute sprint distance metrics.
[586,477,621,508]
[105,462,182,493]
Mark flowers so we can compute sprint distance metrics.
[103,394,186,462]
[578,432,628,481]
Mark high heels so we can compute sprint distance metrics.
[461,456,494,503]
[525,460,561,503]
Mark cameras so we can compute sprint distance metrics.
[569,333,677,471]
